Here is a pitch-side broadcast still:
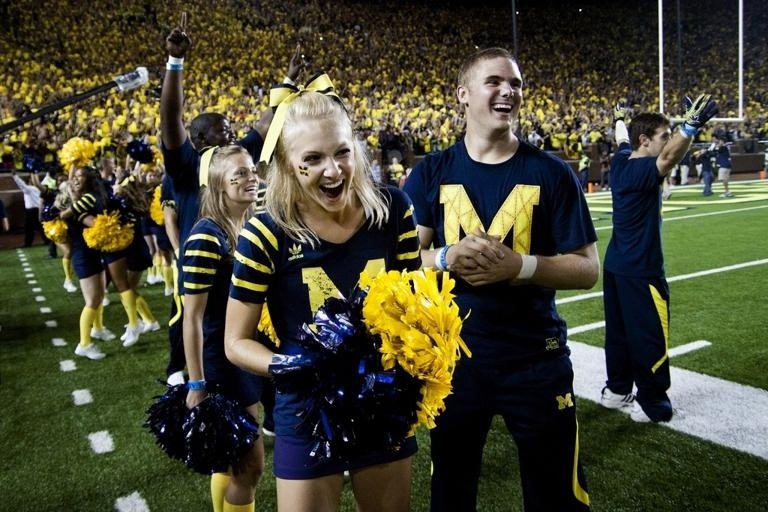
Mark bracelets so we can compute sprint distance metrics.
[165,63,185,71]
[187,380,207,391]
[282,77,295,88]
[517,254,538,280]
[167,54,185,65]
[441,243,453,272]
[435,248,444,273]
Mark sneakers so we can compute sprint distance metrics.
[120,324,129,341]
[103,294,110,306]
[146,275,157,285]
[90,326,116,342]
[63,280,77,292]
[167,371,185,386]
[602,389,636,410]
[262,426,275,437]
[123,319,143,347]
[74,343,106,360]
[142,320,160,333]
[164,287,174,297]
[631,399,685,424]
[155,274,162,283]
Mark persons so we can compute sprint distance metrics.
[225,73,424,512]
[614,0,768,196]
[301,0,467,182]
[174,146,278,512]
[175,0,301,133]
[403,49,591,512]
[467,0,613,190]
[601,94,719,424]
[150,26,304,387]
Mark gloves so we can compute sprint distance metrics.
[612,101,626,122]
[681,94,719,137]
